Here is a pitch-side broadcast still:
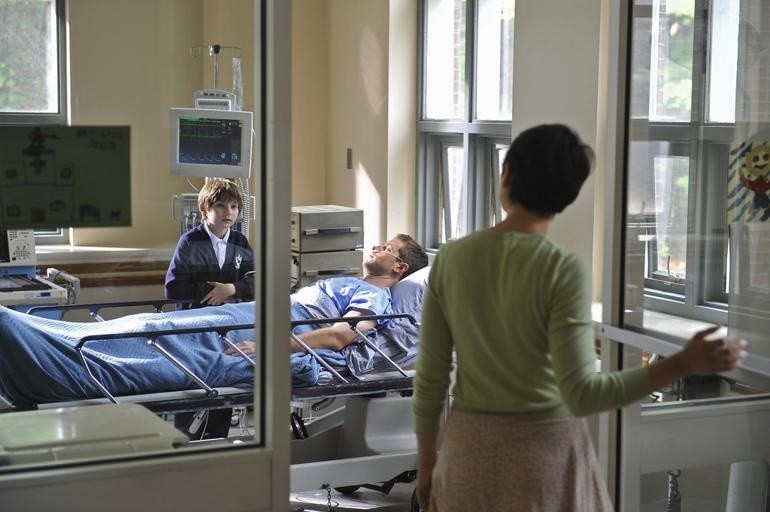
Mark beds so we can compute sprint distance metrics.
[16,246,175,287]
[0,252,461,494]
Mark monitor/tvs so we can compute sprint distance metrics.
[168,107,252,180]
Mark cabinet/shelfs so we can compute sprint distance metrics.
[291,203,365,251]
[289,250,365,291]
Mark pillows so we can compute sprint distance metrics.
[392,266,432,316]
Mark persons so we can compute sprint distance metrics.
[0,233,429,402]
[163,177,255,441]
[412,122,750,511]
